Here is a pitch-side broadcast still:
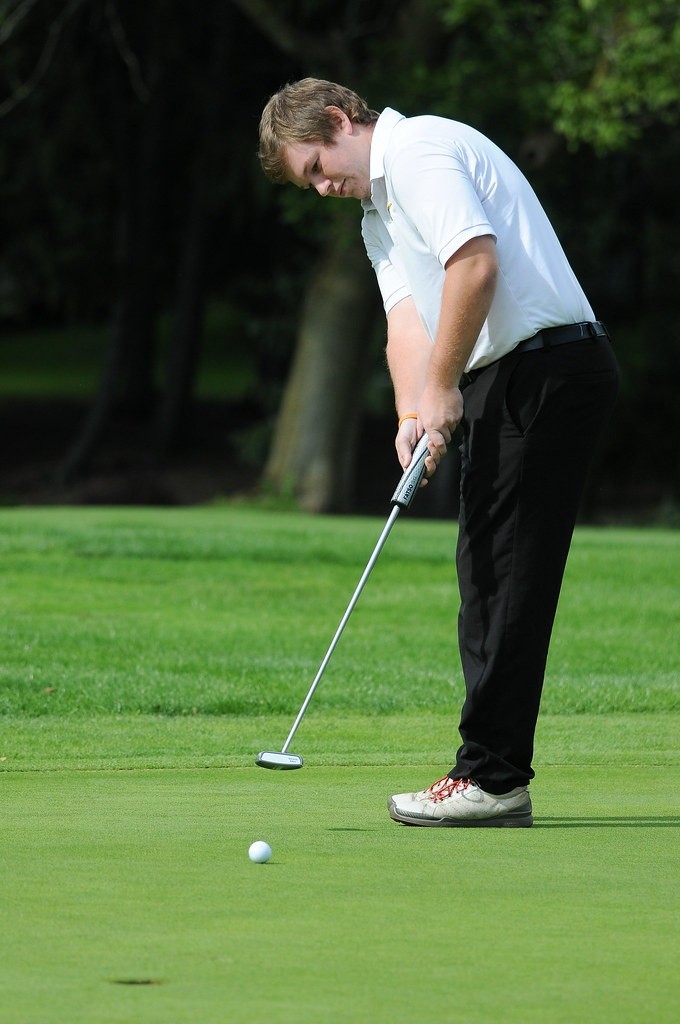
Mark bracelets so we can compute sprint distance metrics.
[398,413,417,428]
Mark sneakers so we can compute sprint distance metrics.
[389,778,536,830]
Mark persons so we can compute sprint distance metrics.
[261,78,619,827]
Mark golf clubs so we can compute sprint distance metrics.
[254,432,431,771]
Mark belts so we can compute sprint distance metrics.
[459,323,607,385]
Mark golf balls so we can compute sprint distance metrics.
[249,840,270,864]
